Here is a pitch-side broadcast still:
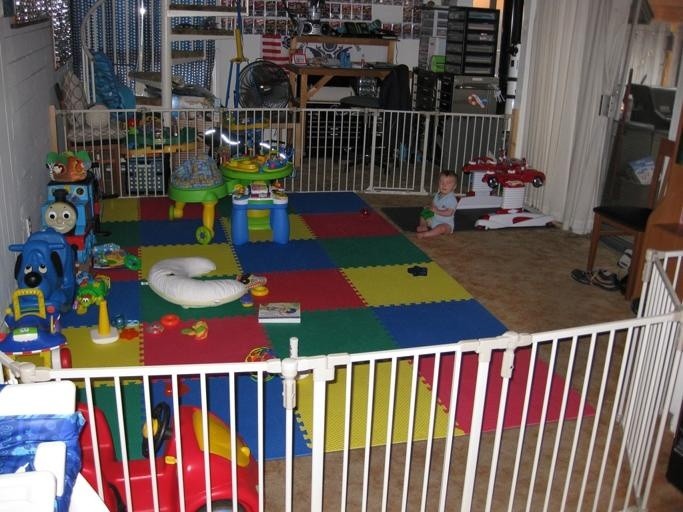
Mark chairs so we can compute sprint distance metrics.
[615,93,655,155]
[588,140,677,285]
[50,83,124,195]
[341,65,411,172]
[252,55,302,166]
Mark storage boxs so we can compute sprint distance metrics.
[126,153,167,194]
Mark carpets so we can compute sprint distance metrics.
[381,206,559,232]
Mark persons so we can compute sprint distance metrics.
[416,170,458,239]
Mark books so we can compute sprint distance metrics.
[258,302,301,324]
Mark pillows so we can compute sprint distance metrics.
[143,256,252,309]
[54,73,90,128]
[67,120,124,141]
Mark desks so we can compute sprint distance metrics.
[291,34,396,185]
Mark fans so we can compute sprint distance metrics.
[237,58,291,109]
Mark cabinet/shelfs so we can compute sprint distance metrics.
[416,6,500,170]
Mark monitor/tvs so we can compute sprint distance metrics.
[623,82,683,165]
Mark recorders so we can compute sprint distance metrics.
[298,20,330,37]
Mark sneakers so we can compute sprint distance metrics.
[571,268,619,292]
